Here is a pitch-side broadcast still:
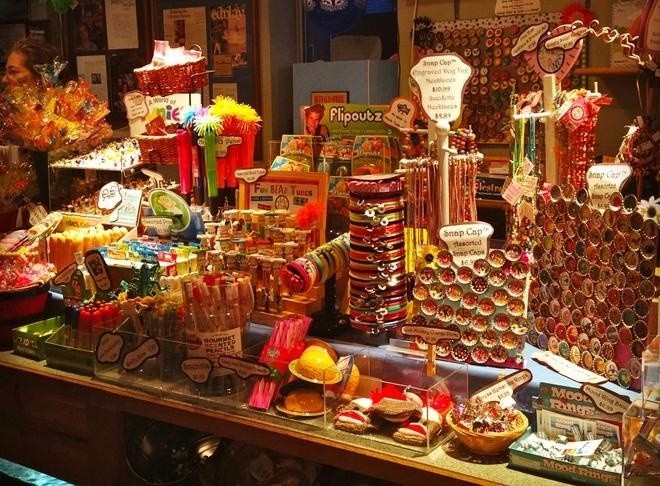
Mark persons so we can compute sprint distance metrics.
[304,104,329,141]
[77,9,103,52]
[114,72,135,112]
[1,37,61,89]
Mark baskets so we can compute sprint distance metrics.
[133,44,209,96]
[136,131,179,165]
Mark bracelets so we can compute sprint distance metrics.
[281,232,350,295]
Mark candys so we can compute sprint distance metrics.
[0,158,36,207]
[452,400,518,434]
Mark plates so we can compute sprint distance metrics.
[275,402,330,419]
[287,357,344,385]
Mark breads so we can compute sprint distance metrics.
[284,339,360,413]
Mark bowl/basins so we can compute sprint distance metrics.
[0,280,56,320]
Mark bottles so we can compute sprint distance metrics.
[63,296,123,352]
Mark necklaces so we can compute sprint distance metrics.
[400,157,478,246]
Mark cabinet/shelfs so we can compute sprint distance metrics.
[0,312,643,485]
